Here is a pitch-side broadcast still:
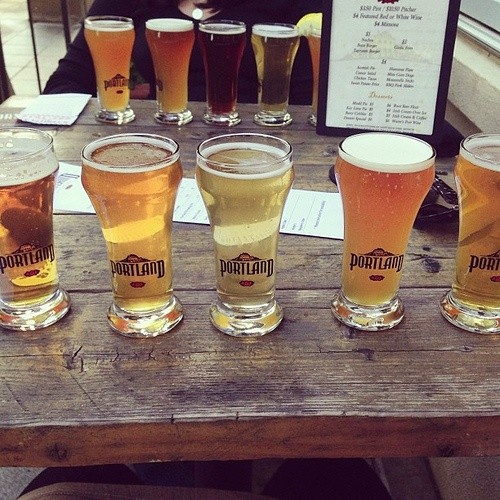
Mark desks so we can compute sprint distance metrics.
[0,95,500,500]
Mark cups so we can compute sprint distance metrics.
[439,132,500,334]
[193,132,296,338]
[306,23,321,125]
[80,132,185,339]
[0,126,72,333]
[250,22,302,127]
[144,17,195,126]
[197,19,247,128]
[329,133,435,331]
[82,15,136,124]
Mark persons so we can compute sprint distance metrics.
[19,459,392,500]
[41,0,321,107]
